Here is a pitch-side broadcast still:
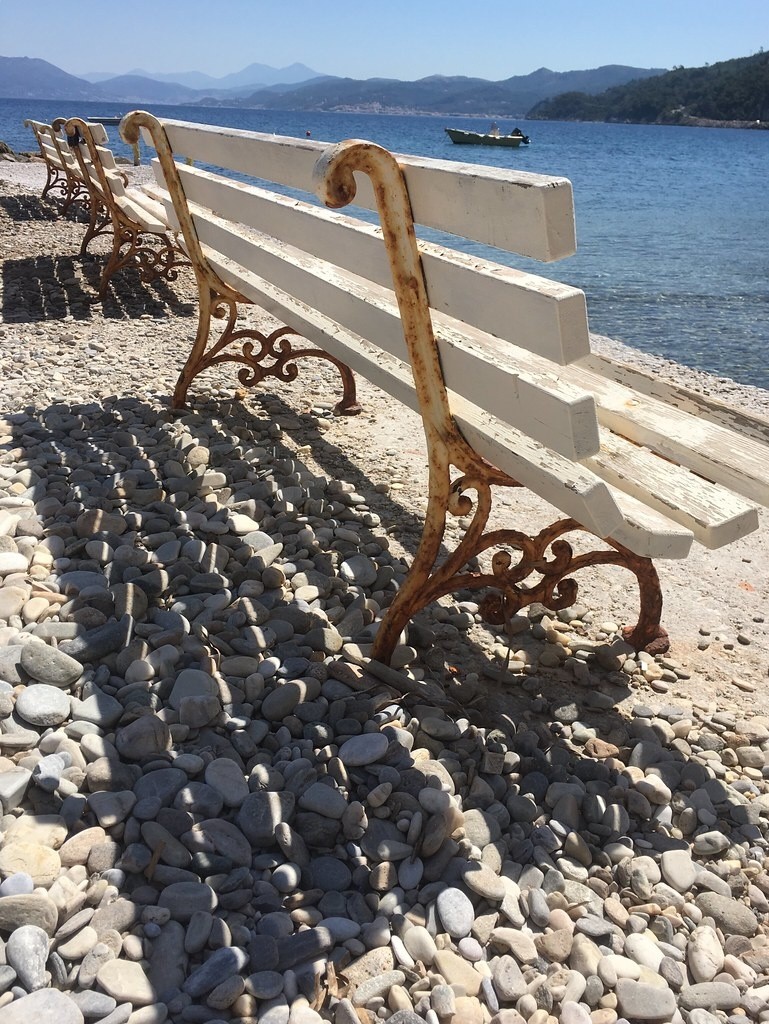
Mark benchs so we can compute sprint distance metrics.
[24,117,216,305]
[121,110,768,664]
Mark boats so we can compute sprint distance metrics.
[87,115,121,126]
[445,122,532,147]
